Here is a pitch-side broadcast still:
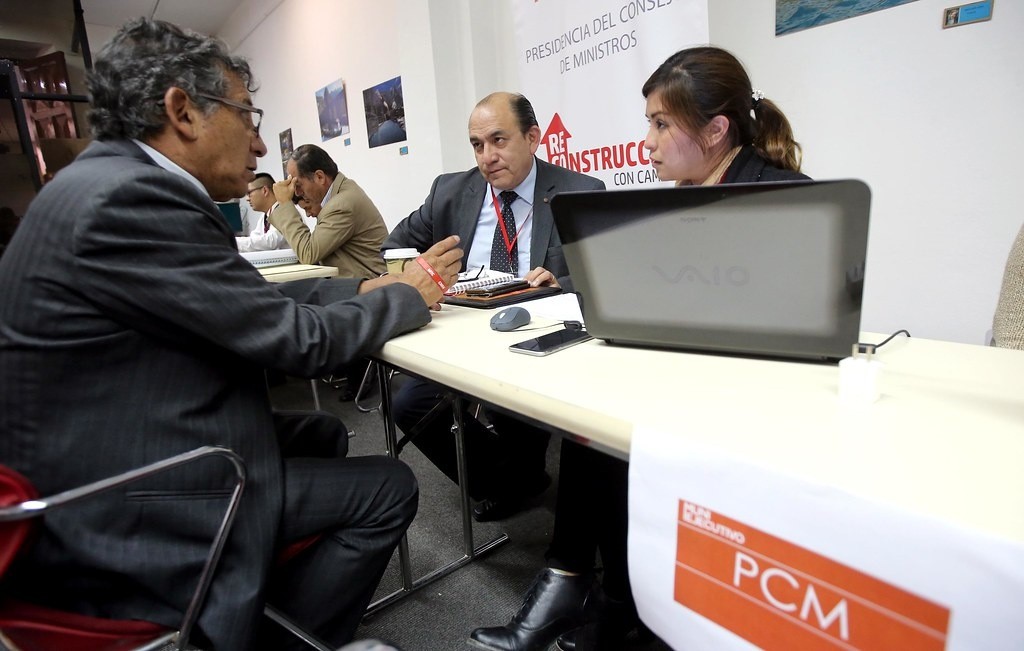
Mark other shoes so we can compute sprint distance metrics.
[340,382,364,401]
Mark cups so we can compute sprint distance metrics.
[382,248,420,276]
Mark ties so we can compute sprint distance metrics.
[490,192,520,278]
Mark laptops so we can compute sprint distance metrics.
[550,179,872,365]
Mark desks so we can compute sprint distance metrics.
[354,276,1024,651]
[253,259,360,442]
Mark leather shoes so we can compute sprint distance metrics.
[556,596,664,651]
[473,476,553,521]
[466,571,607,651]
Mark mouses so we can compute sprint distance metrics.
[489,307,530,332]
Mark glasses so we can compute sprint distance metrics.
[247,187,271,196]
[157,92,264,135]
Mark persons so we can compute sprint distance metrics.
[468,45,816,651]
[234,173,304,253]
[380,90,608,527]
[1,13,420,651]
[266,144,392,408]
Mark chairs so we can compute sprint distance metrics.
[1,432,247,651]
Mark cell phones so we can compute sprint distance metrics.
[509,329,594,357]
